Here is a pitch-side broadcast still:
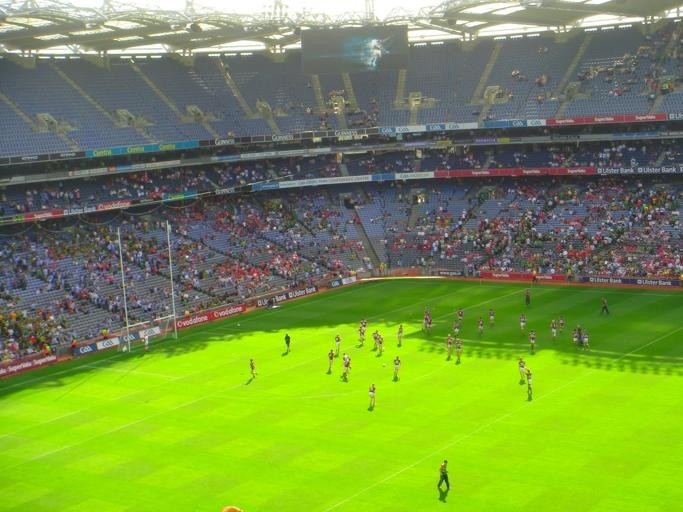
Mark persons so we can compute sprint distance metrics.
[470,87,514,121]
[0,129,683,410]
[437,460,451,490]
[604,25,682,102]
[509,47,604,104]
[307,90,380,130]
